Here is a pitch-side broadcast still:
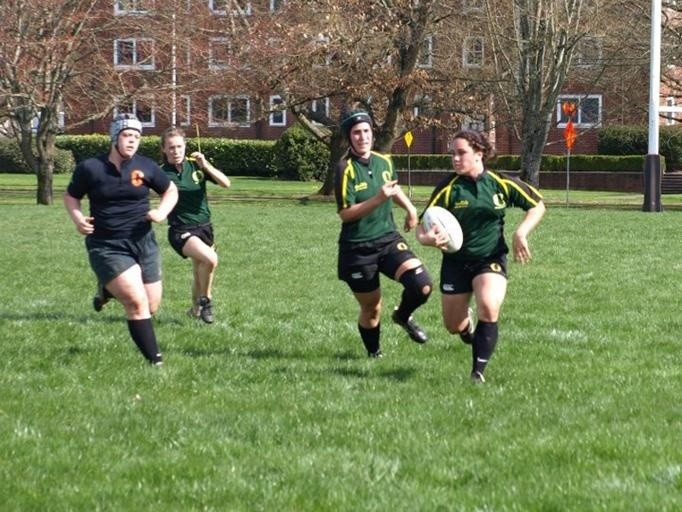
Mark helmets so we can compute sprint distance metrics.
[341,108,373,136]
[112,112,144,145]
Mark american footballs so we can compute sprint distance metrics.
[423,206,463,253]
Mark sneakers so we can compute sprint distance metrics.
[365,350,386,362]
[470,368,485,387]
[460,309,476,344]
[149,358,168,374]
[93,280,110,312]
[391,308,427,343]
[200,296,213,323]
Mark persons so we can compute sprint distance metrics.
[63,113,179,365]
[157,126,230,323]
[335,109,433,360]
[416,131,546,384]
